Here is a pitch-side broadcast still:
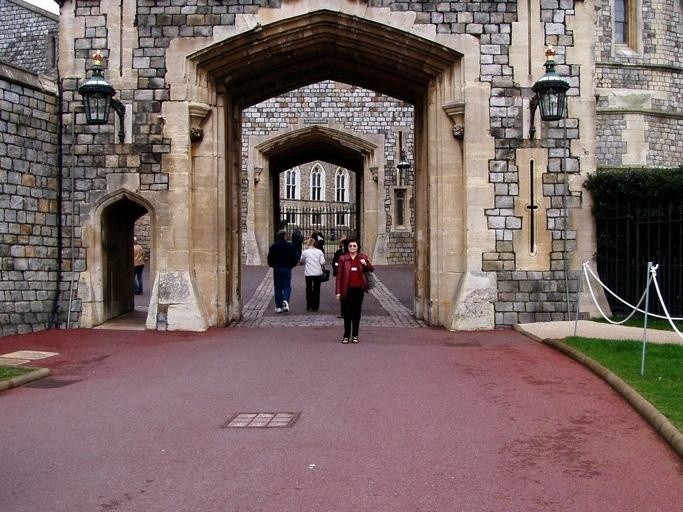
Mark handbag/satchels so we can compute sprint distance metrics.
[365,270,375,289]
[321,266,330,282]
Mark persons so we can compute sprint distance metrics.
[334,237,375,344]
[132,236,145,295]
[291,225,304,262]
[331,234,349,318]
[312,228,324,255]
[266,229,299,314]
[298,237,326,313]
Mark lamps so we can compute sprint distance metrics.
[524,48,568,142]
[76,49,124,142]
[396,148,411,186]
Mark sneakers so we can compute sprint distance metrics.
[273,300,290,314]
[341,337,358,344]
